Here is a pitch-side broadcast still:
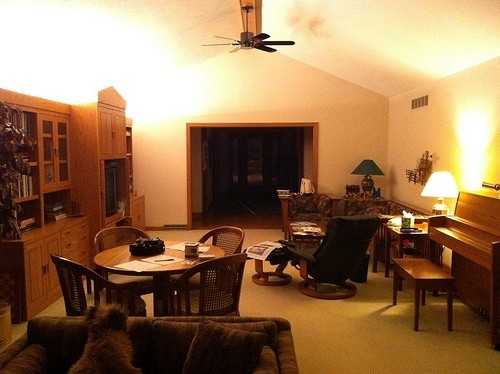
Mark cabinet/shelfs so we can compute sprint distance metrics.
[0,88,145,322]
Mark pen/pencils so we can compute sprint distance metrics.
[155,259,174,262]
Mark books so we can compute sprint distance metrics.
[16,173,67,232]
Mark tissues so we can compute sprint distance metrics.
[401,209,415,228]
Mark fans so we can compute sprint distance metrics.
[202,5,295,53]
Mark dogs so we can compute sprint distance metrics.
[67,303,142,374]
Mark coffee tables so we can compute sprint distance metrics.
[288,220,327,249]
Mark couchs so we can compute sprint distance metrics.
[279,190,331,240]
[352,194,426,273]
[0,315,298,374]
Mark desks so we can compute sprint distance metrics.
[383,222,429,290]
[343,193,390,217]
[94,240,225,317]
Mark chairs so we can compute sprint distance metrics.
[287,214,380,299]
[49,226,247,317]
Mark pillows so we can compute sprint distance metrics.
[184,316,268,374]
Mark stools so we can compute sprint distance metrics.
[243,240,296,285]
[392,258,458,331]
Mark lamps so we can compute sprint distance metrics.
[351,160,385,194]
[421,171,459,216]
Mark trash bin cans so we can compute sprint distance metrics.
[0,301,12,347]
